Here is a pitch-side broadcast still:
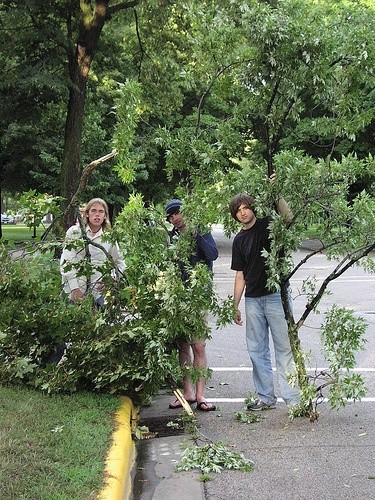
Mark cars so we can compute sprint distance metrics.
[0,214,23,224]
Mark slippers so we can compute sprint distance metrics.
[197,401,216,411]
[168,397,197,409]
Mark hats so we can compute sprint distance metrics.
[164,199,183,211]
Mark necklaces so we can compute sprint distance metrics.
[92,227,102,233]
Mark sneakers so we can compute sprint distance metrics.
[247,398,277,411]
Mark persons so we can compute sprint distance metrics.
[46,198,127,369]
[161,200,219,410]
[228,173,308,410]
